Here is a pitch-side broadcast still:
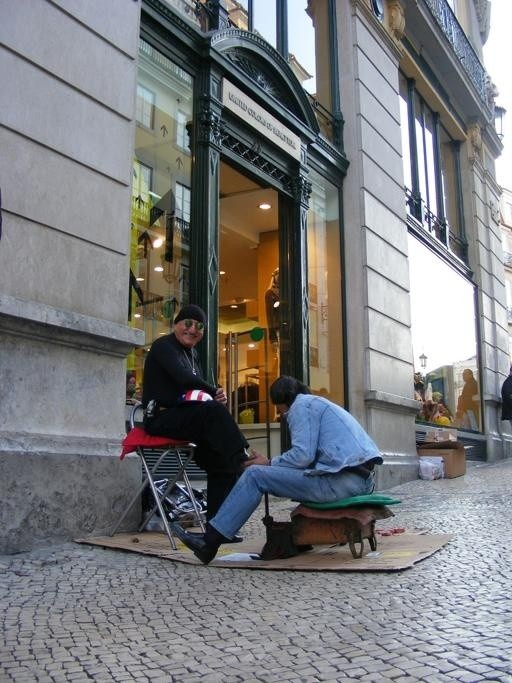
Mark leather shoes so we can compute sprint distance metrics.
[227,448,253,474]
[174,521,244,564]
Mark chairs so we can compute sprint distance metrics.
[127,402,253,553]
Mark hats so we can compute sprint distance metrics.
[174,304,206,325]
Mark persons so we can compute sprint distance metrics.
[499,366,511,424]
[454,367,480,432]
[170,374,384,567]
[146,303,267,543]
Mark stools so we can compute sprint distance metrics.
[290,495,389,561]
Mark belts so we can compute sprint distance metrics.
[348,467,369,479]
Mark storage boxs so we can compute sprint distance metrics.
[418,428,467,481]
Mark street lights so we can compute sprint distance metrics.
[419,351,427,378]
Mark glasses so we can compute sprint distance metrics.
[183,318,204,329]
